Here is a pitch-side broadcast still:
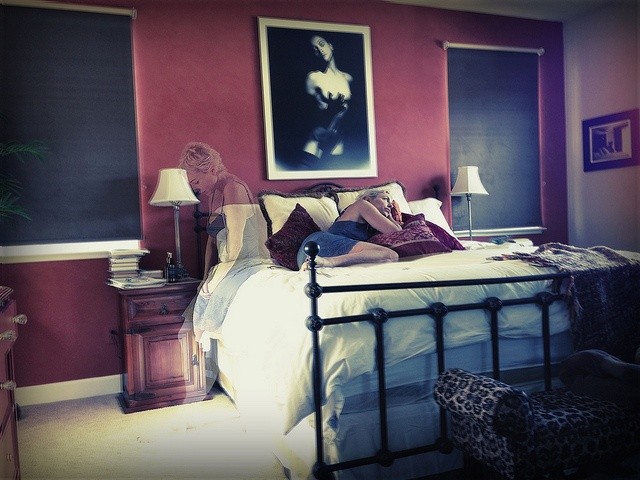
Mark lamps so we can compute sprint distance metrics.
[148,169,200,278]
[450,166,489,240]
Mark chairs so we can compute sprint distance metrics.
[435,352,640,479]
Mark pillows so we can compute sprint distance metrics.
[264,202,321,271]
[331,181,413,217]
[258,193,340,239]
[366,213,452,258]
[408,197,460,243]
[215,204,270,262]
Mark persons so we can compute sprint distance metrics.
[298,35,355,170]
[297,187,403,271]
[175,140,272,431]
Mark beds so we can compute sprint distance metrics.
[191,182,640,480]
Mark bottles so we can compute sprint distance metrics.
[165,252,178,285]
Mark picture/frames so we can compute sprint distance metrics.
[582,109,640,172]
[258,17,378,179]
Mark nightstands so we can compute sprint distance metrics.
[118,279,212,414]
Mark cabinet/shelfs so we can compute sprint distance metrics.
[0,287,28,480]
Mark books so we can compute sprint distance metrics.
[109,248,167,290]
